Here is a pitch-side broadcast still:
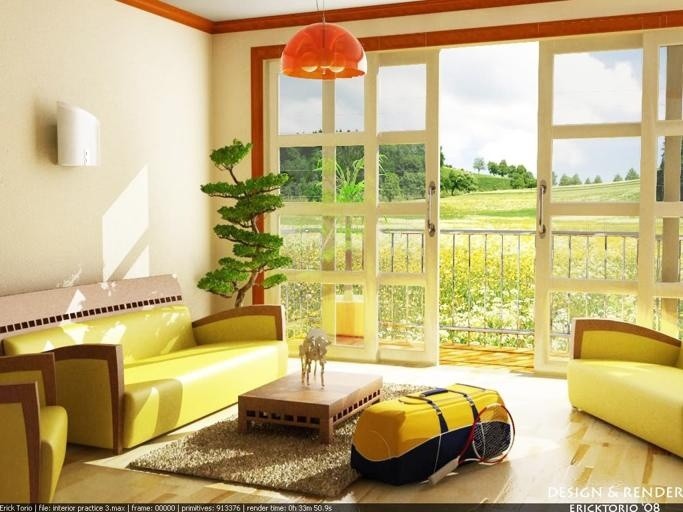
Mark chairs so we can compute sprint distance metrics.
[0,352,69,504]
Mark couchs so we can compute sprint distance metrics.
[565,316,683,459]
[2,304,291,456]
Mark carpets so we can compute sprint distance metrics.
[126,385,437,498]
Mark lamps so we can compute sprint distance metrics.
[55,99,102,168]
[279,0,368,80]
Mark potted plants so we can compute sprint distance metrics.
[312,153,392,338]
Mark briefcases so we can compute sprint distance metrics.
[350,382,507,486]
